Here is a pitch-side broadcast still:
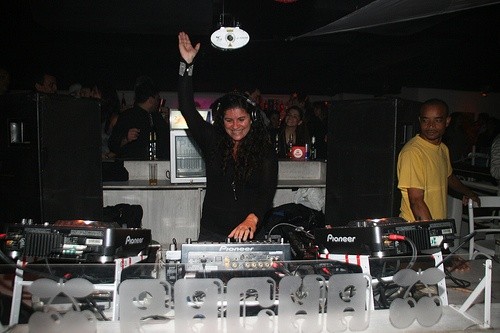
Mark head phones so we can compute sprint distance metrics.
[216,92,260,122]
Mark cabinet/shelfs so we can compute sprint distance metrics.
[102,160,326,250]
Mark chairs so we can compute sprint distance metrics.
[468,196,500,261]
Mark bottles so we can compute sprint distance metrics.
[273,133,280,158]
[310,135,317,160]
[288,134,294,159]
[257,98,289,120]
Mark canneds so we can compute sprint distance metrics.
[304,143,309,159]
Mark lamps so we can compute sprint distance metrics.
[208,0,251,52]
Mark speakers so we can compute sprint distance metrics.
[326,96,423,226]
[0,89,104,226]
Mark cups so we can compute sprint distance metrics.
[149,163,157,184]
[10,122,23,143]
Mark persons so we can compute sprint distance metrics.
[178,32,278,243]
[0,67,170,180]
[397,97,480,273]
[442,111,500,185]
[245,89,328,159]
